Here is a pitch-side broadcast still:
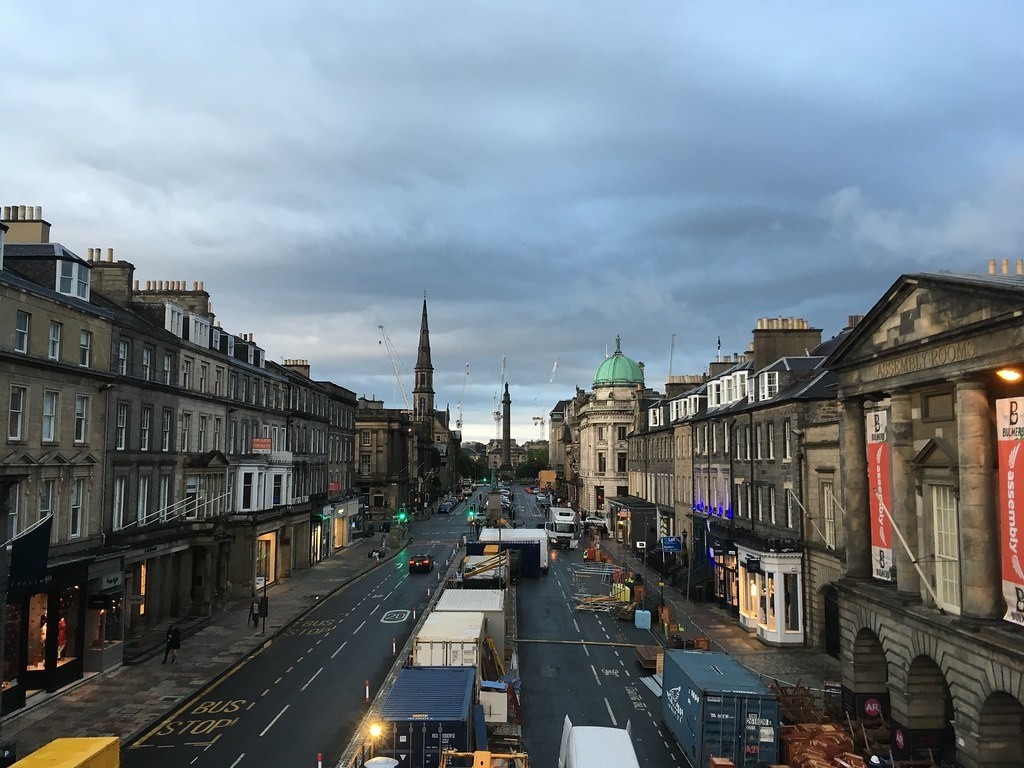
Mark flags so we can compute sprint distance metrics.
[718,336,720,350]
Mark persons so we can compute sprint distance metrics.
[382,534,386,551]
[161,625,181,665]
[579,510,608,541]
[523,522,526,529]
[249,598,261,629]
[371,549,379,561]
[508,505,517,521]
[761,586,791,624]
[581,547,588,562]
[108,606,117,643]
[751,580,756,611]
[402,525,406,539]
[40,610,66,666]
[545,488,562,507]
[513,521,517,529]
[636,572,643,584]
[719,567,725,593]
[544,507,548,519]
[465,496,468,503]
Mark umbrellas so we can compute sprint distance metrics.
[248,613,251,625]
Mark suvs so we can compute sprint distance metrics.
[409,554,434,573]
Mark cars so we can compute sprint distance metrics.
[531,484,550,508]
[438,486,472,514]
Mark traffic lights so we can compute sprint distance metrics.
[469,505,474,516]
[399,508,405,521]
[484,477,487,482]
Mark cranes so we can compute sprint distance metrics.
[456,361,470,431]
[378,323,415,410]
[533,358,560,440]
[493,353,507,440]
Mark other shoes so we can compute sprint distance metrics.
[171,658,174,663]
[162,660,166,664]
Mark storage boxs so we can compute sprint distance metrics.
[462,556,506,589]
[479,528,548,568]
[436,588,504,663]
[412,612,485,702]
[663,650,779,768]
[373,666,476,768]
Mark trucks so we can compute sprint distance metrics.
[545,507,578,550]
